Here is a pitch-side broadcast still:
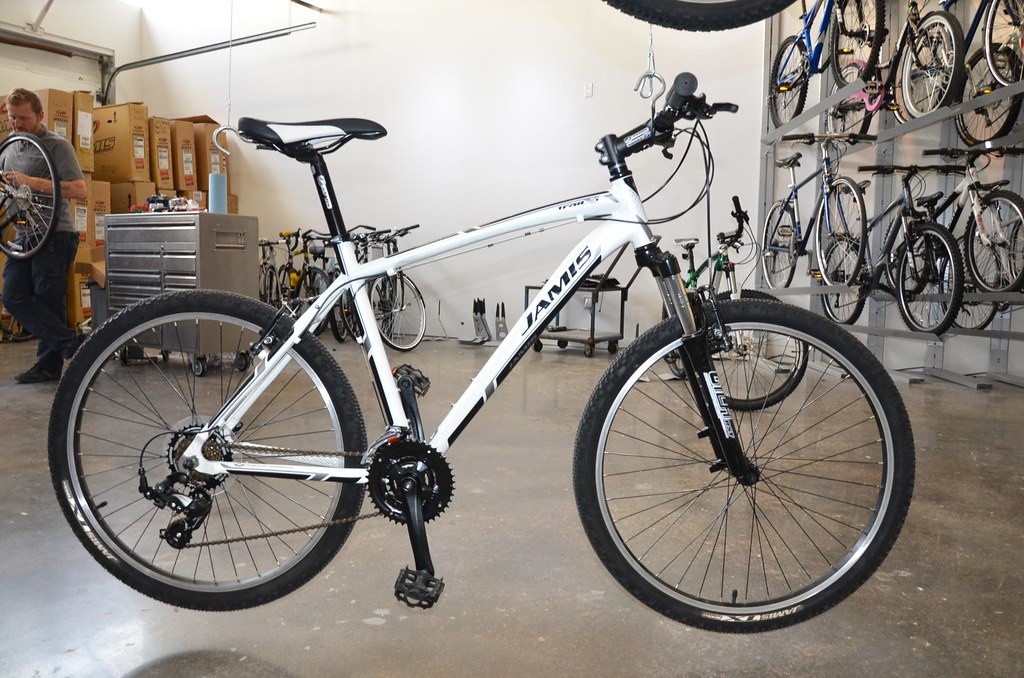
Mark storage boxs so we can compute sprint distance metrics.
[0,88,239,332]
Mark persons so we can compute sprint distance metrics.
[1,89,89,382]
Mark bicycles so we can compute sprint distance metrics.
[255,223,428,352]
[0,133,62,263]
[662,194,810,409]
[603,0,1024,334]
[47,68,917,633]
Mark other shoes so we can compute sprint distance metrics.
[14,358,61,382]
[67,332,90,360]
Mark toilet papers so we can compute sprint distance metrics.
[208,173,227,214]
